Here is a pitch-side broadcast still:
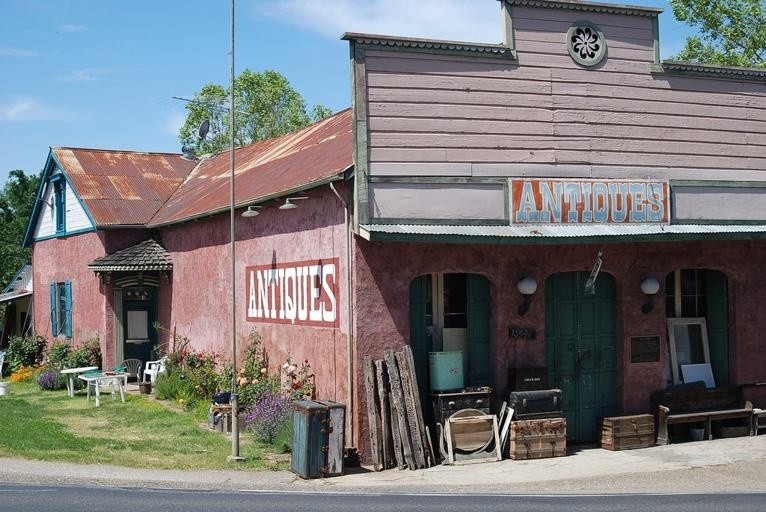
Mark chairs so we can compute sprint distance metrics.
[121,359,141,384]
[143,359,165,393]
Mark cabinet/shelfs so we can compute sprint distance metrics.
[430,390,492,454]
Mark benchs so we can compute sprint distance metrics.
[650,381,766,445]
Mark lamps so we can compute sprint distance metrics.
[518,277,537,315]
[279,195,323,209]
[242,204,274,217]
[641,278,659,312]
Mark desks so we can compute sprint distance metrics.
[61,367,98,397]
[78,373,127,407]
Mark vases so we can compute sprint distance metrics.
[140,383,151,394]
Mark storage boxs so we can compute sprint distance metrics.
[597,413,656,450]
[508,366,568,460]
